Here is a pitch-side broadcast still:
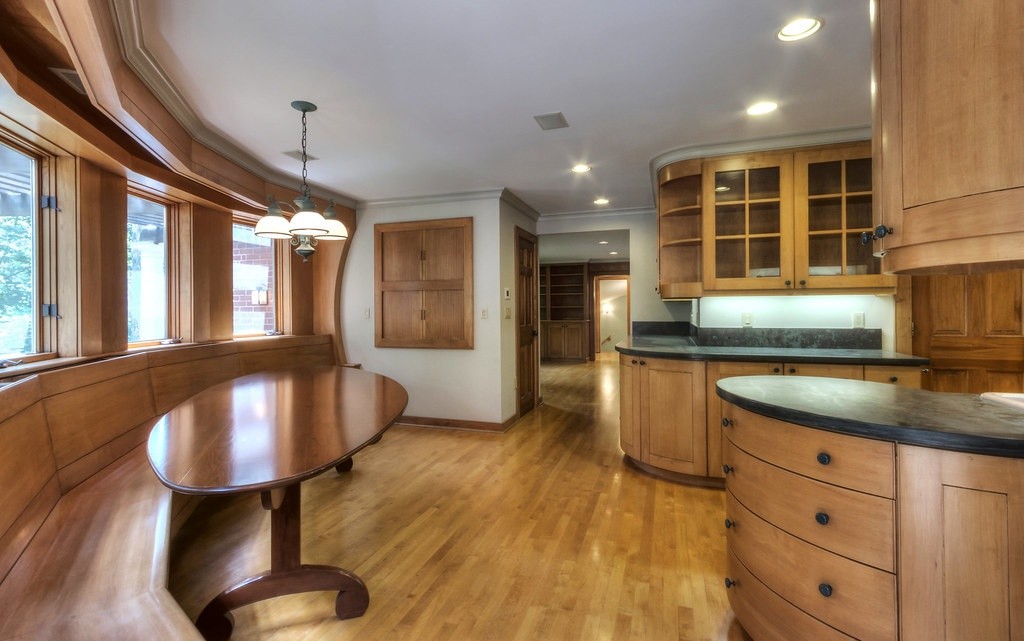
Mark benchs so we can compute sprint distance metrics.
[0,334,361,641]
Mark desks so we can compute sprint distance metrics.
[146,364,408,641]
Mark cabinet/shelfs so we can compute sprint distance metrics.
[540,261,591,363]
[618,353,923,488]
[374,217,474,349]
[702,146,897,297]
[872,0,1024,272]
[719,396,1024,641]
[655,159,704,299]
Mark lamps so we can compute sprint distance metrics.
[254,101,349,263]
